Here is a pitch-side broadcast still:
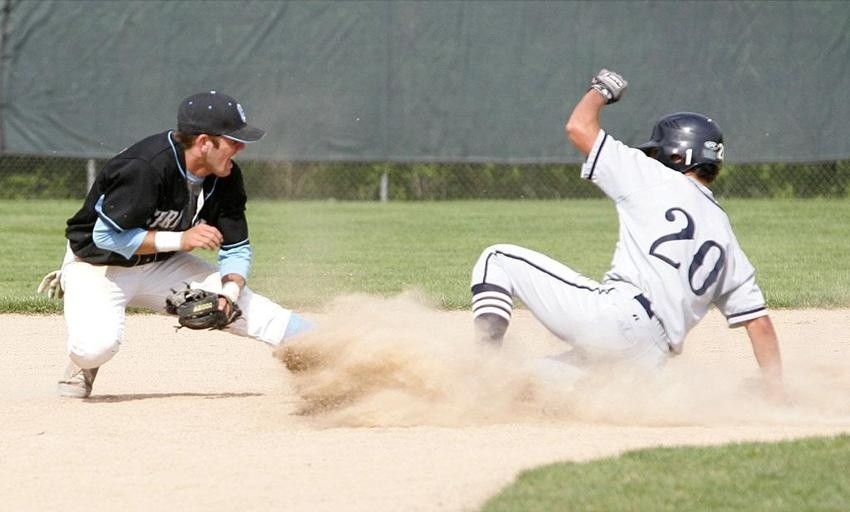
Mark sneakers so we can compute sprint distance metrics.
[59,359,99,397]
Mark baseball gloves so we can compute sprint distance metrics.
[166,281,243,331]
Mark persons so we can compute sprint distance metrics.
[470,69,785,386]
[57,91,314,397]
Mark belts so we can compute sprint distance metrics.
[635,293,655,319]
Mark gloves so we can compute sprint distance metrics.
[38,270,63,300]
[589,69,627,104]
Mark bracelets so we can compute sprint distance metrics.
[155,231,182,254]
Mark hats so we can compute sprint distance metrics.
[176,92,266,144]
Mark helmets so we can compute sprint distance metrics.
[635,112,722,174]
